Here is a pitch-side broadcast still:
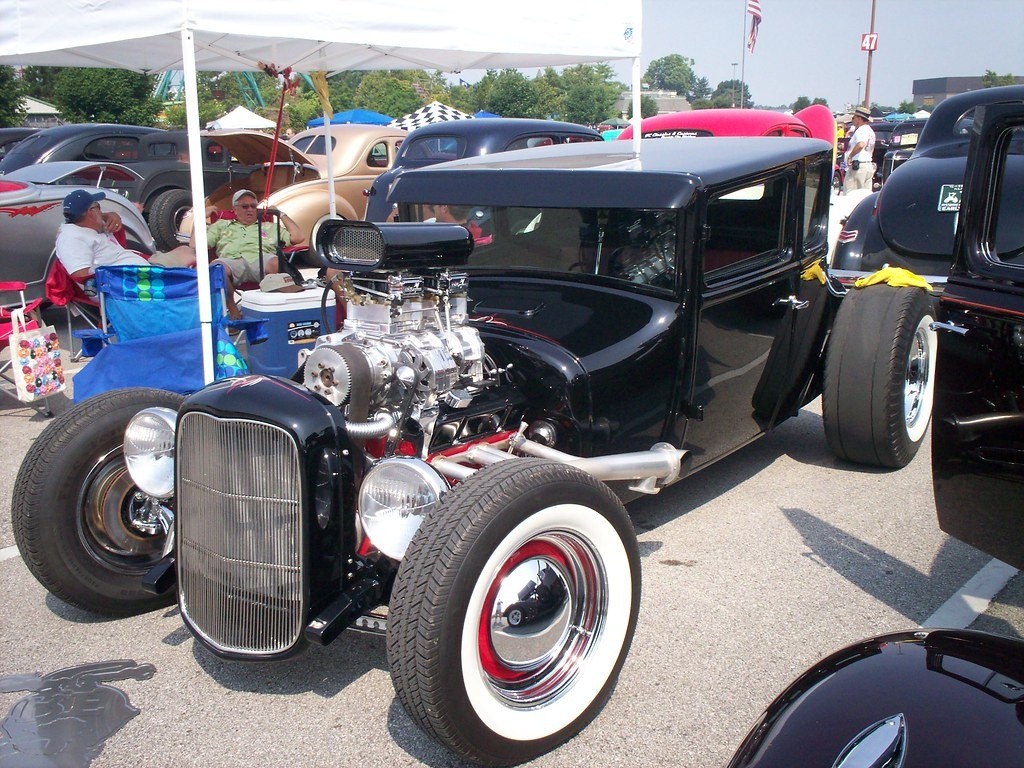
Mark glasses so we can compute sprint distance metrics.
[235,203,258,209]
[287,133,293,135]
[91,204,101,210]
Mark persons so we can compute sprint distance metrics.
[843,107,875,196]
[435,204,482,239]
[415,205,436,223]
[55,189,305,336]
[586,122,614,134]
[285,128,296,139]
[837,124,845,138]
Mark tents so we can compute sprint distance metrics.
[473,110,502,118]
[600,117,630,129]
[306,109,394,130]
[885,110,930,121]
[0,0,641,385]
[386,100,476,131]
[205,106,276,129]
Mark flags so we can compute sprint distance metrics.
[747,0,761,53]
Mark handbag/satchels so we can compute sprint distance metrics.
[8,306,68,404]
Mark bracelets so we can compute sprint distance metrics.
[280,212,286,219]
[848,154,850,159]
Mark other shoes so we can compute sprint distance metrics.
[229,329,241,336]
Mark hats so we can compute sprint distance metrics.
[837,124,842,126]
[854,107,873,123]
[232,189,259,206]
[62,189,106,224]
[259,273,311,293]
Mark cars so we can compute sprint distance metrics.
[726,85,1024,768]
[0,105,835,308]
[10,137,937,768]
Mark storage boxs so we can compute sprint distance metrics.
[226,285,337,379]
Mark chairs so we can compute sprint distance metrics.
[0,206,311,421]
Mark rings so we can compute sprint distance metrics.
[115,224,119,228]
